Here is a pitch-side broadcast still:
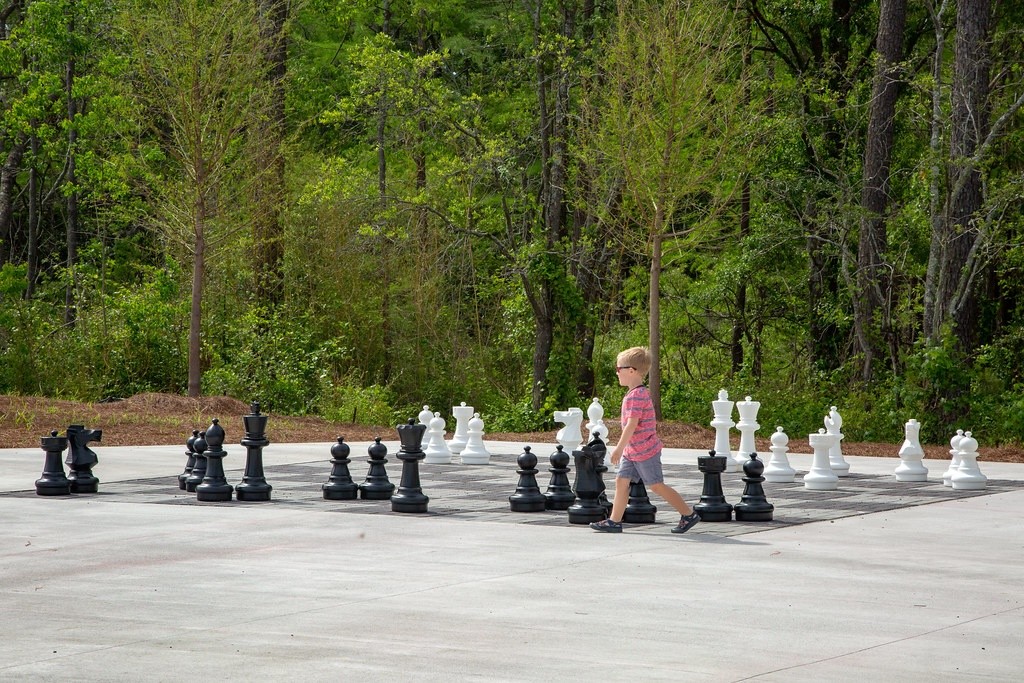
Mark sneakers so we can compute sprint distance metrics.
[590,518,622,532]
[671,511,701,533]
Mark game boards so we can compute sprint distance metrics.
[1,449,1024,540]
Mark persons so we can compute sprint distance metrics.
[589,345,700,533]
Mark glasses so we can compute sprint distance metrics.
[616,366,637,373]
